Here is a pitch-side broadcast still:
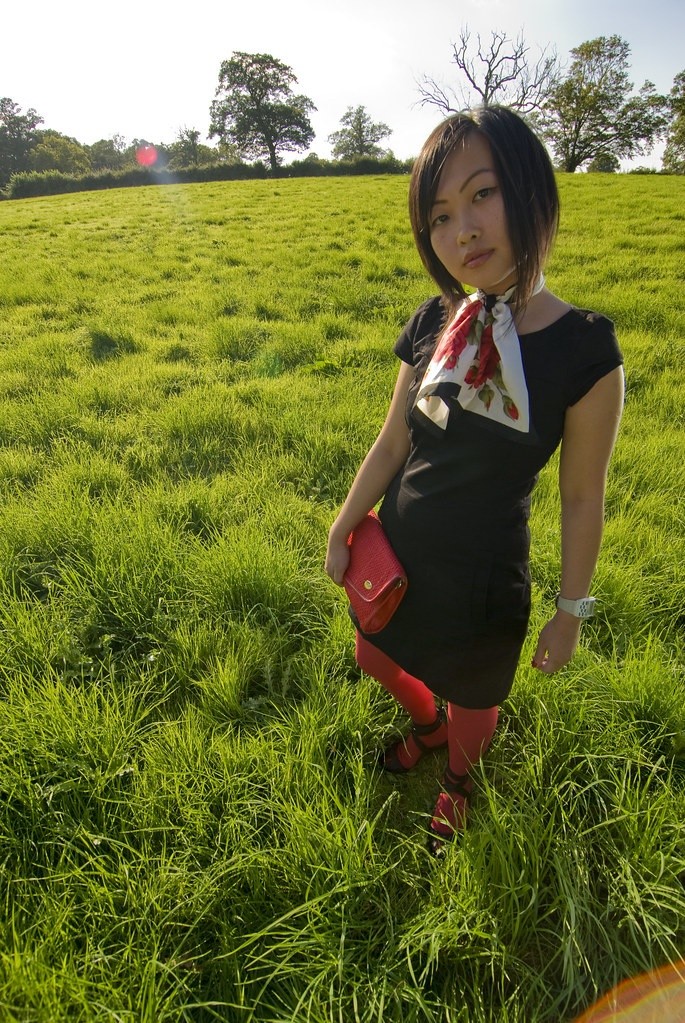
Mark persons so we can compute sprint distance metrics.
[323,106,624,854]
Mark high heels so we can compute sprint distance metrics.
[380,714,447,772]
[427,766,476,858]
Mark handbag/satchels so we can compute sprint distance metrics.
[343,507,407,634]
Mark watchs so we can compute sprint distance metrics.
[555,594,597,617]
